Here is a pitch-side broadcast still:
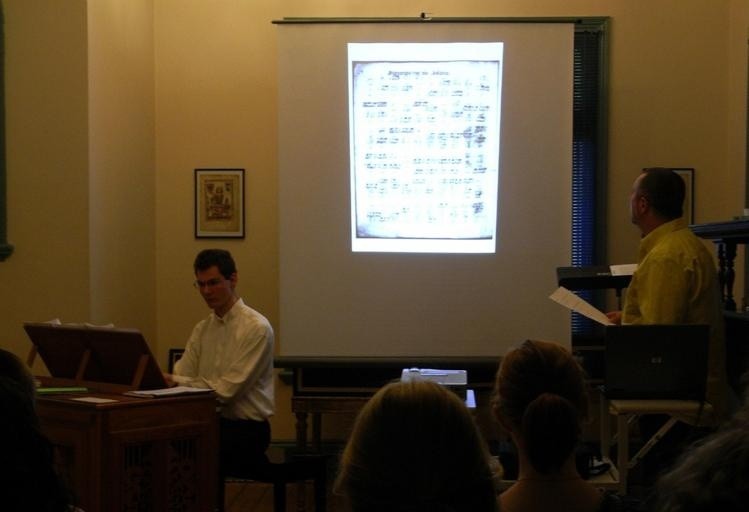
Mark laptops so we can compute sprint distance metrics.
[605,324,711,400]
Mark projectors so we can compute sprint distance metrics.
[401,368,468,402]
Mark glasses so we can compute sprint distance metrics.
[191,274,226,290]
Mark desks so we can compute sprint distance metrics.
[598,386,713,496]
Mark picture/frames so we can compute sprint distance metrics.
[641,167,695,227]
[194,168,246,240]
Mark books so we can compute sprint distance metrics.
[124,386,217,398]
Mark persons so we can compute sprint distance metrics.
[604,165,729,510]
[164,248,275,511]
[491,338,625,511]
[0,349,84,512]
[644,412,748,511]
[331,377,503,511]
